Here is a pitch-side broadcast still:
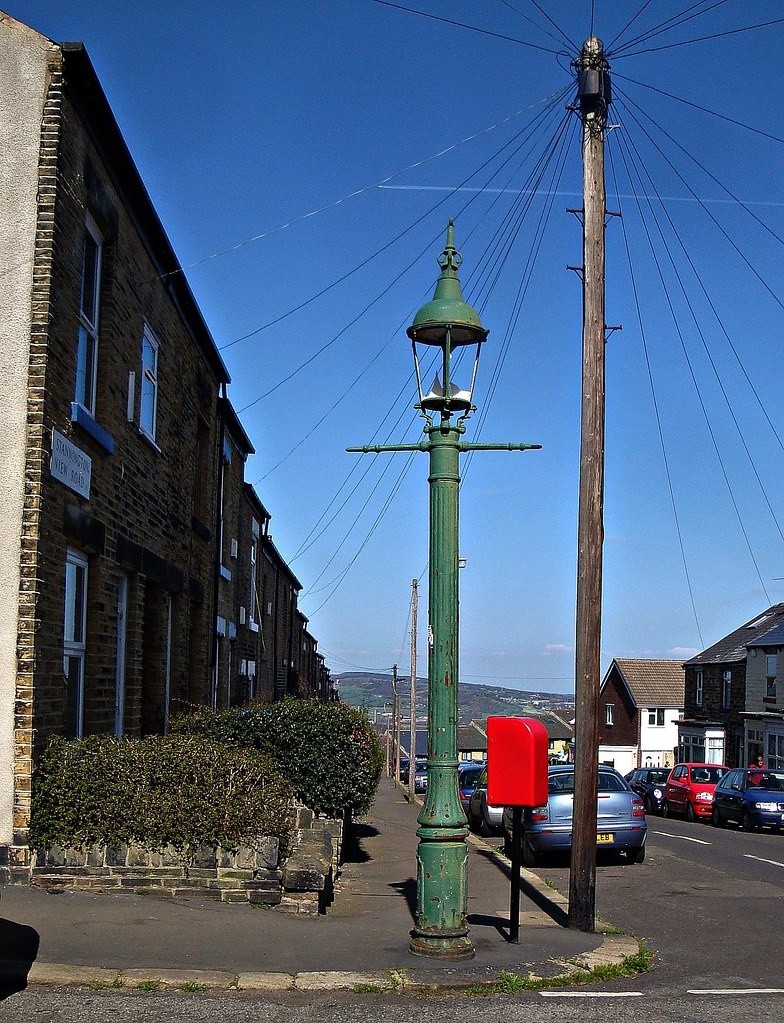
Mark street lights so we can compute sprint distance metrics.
[342,215,542,961]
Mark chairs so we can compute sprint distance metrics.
[699,772,708,782]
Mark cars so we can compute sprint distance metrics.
[518,764,648,869]
[391,754,545,838]
[710,767,784,833]
[660,762,733,822]
[627,767,674,815]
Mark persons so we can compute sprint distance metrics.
[747,756,766,786]
